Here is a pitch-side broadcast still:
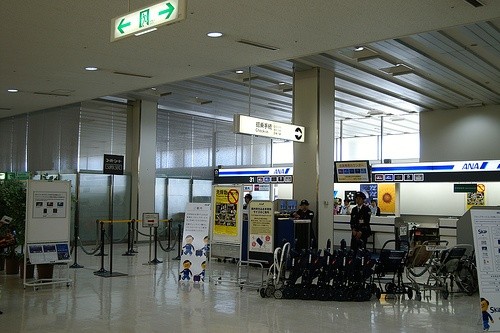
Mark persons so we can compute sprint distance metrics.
[243,192,380,250]
[350,192,373,250]
[290,200,317,247]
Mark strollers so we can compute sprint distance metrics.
[260,240,478,304]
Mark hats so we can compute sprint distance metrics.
[355,192,366,199]
[244,194,252,198]
[300,200,309,206]
